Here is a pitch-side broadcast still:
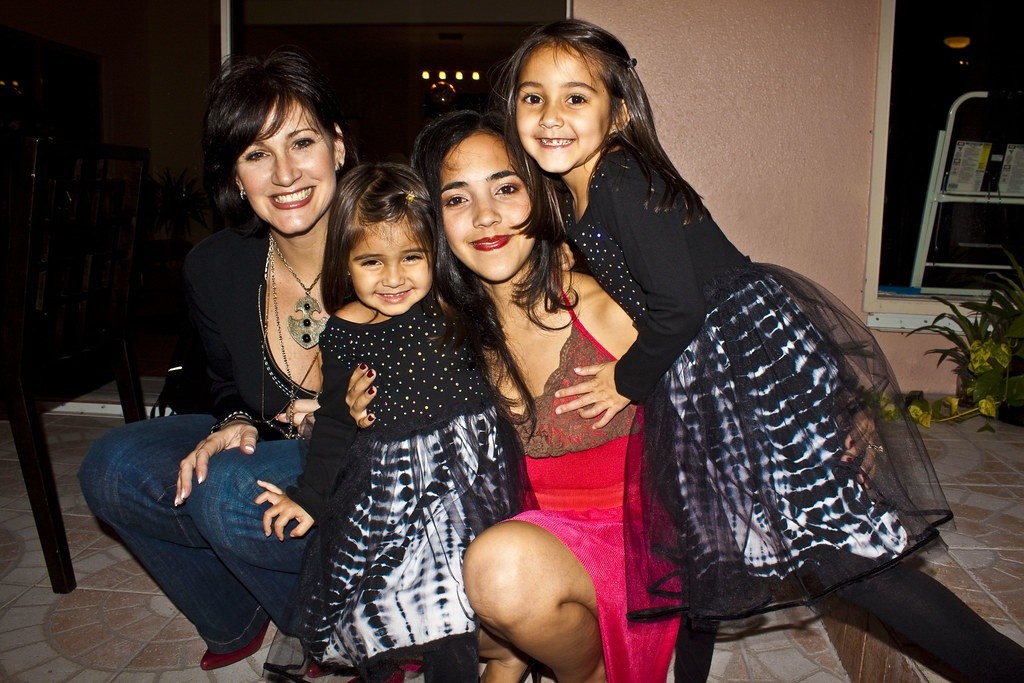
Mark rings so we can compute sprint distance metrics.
[866,443,883,454]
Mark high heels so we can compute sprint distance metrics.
[306,658,332,678]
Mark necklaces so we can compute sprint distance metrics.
[261,234,329,439]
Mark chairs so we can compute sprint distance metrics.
[0,134,154,594]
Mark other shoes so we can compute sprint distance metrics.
[520,654,542,683]
[200,614,272,671]
[346,666,405,683]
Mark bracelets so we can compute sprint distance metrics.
[210,411,255,434]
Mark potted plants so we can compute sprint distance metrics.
[860,246,1024,432]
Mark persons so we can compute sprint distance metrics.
[77,43,357,682]
[500,19,1024,682]
[255,160,541,683]
[346,108,883,683]
